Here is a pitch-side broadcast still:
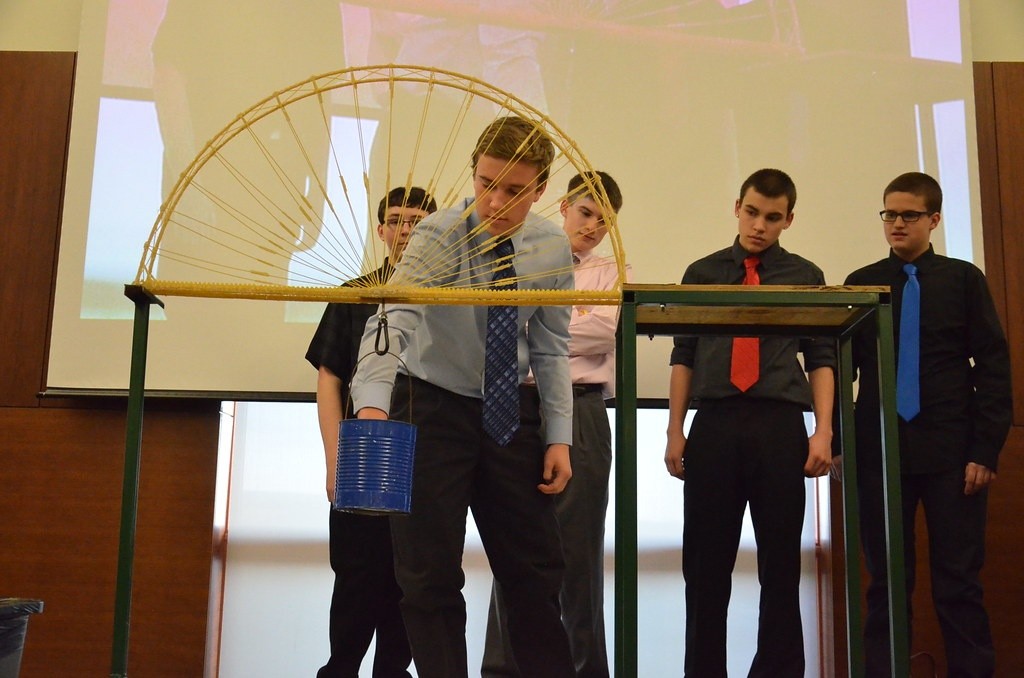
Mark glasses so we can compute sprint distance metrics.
[880,210,931,222]
[382,218,419,226]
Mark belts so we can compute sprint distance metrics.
[521,384,601,397]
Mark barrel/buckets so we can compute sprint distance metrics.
[334,349,415,520]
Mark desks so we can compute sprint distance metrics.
[615,284,912,678]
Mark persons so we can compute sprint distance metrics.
[829,171,1013,678]
[480,171,626,678]
[349,116,576,677]
[306,187,436,678]
[664,169,838,678]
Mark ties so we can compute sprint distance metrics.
[895,263,921,424]
[729,256,762,393]
[480,237,521,450]
